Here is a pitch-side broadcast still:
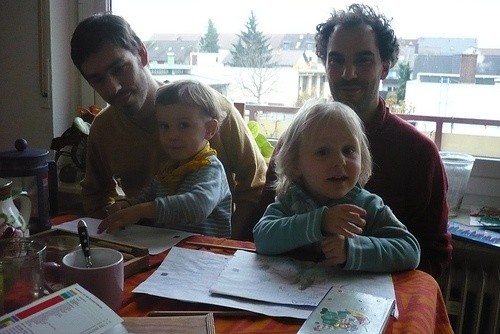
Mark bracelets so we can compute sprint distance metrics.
[117,198,131,205]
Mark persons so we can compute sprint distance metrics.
[252,97,421,271]
[70,12,268,234]
[96,81,232,238]
[251,5,448,279]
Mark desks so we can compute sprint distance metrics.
[0,211,453,334]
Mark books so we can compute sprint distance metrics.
[0,283,128,334]
[297,287,395,334]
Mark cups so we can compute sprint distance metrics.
[439,152,475,218]
[62,249,125,313]
[0,139,59,233]
[0,180,33,238]
[0,238,60,311]
